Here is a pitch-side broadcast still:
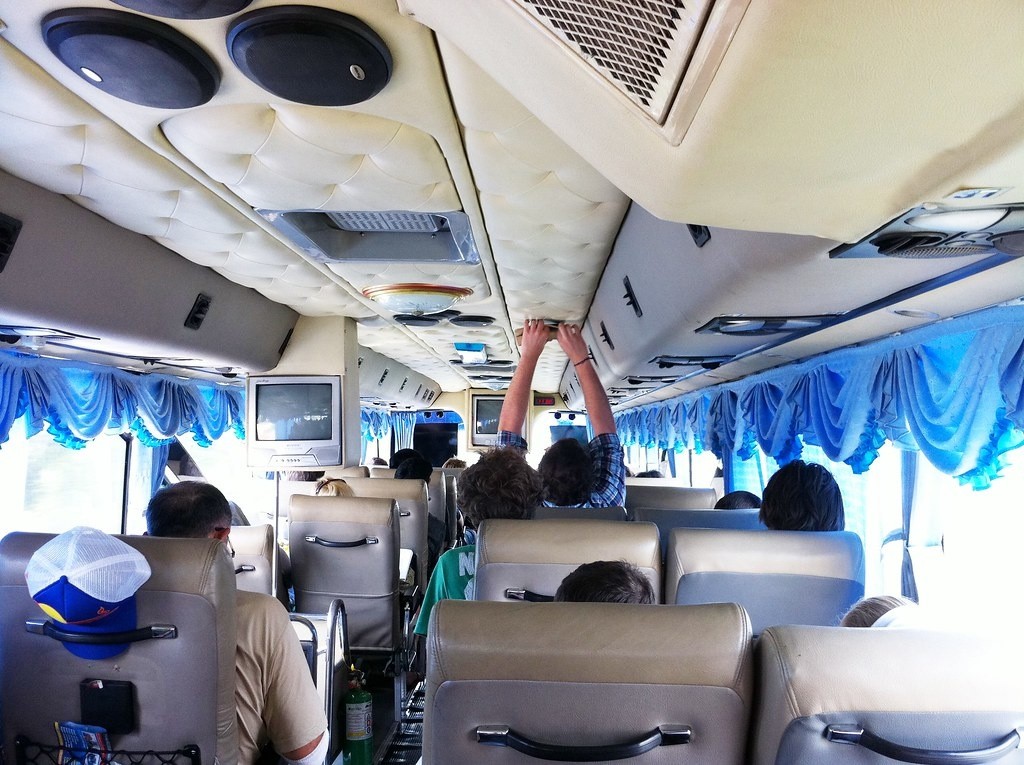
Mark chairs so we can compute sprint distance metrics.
[0,465,1023,765]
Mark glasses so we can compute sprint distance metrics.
[215,526,235,558]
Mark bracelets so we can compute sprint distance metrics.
[574,357,590,366]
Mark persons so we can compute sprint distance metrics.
[714,491,762,509]
[313,476,354,497]
[760,459,845,532]
[496,319,626,508]
[554,560,656,605]
[143,481,330,765]
[369,449,467,579]
[838,595,918,628]
[413,449,545,637]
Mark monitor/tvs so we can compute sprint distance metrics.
[248,376,342,466]
[472,395,526,447]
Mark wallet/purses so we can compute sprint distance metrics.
[80,679,137,735]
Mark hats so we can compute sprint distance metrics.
[24,526,152,660]
